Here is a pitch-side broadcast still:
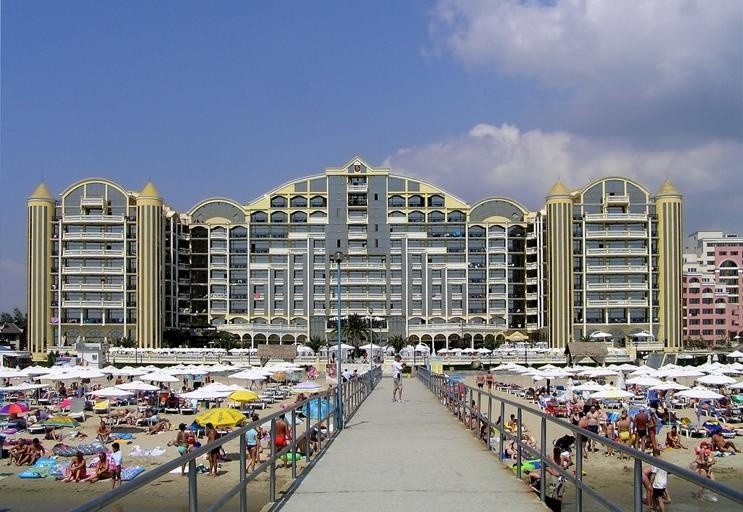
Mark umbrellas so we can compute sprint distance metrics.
[590,330,612,338]
[492,350,742,371]
[628,331,655,341]
[436,347,492,356]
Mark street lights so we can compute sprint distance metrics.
[368,307,373,369]
[334,251,344,428]
[379,324,382,357]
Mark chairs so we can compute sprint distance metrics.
[0,383,224,433]
[490,379,743,458]
[227,383,294,416]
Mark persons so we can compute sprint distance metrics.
[390,354,406,403]
[442,371,743,512]
[0,363,358,488]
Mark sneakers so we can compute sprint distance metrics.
[391,398,403,402]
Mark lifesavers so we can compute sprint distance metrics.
[510,463,534,470]
[527,459,541,469]
[280,452,301,461]
[505,423,526,432]
